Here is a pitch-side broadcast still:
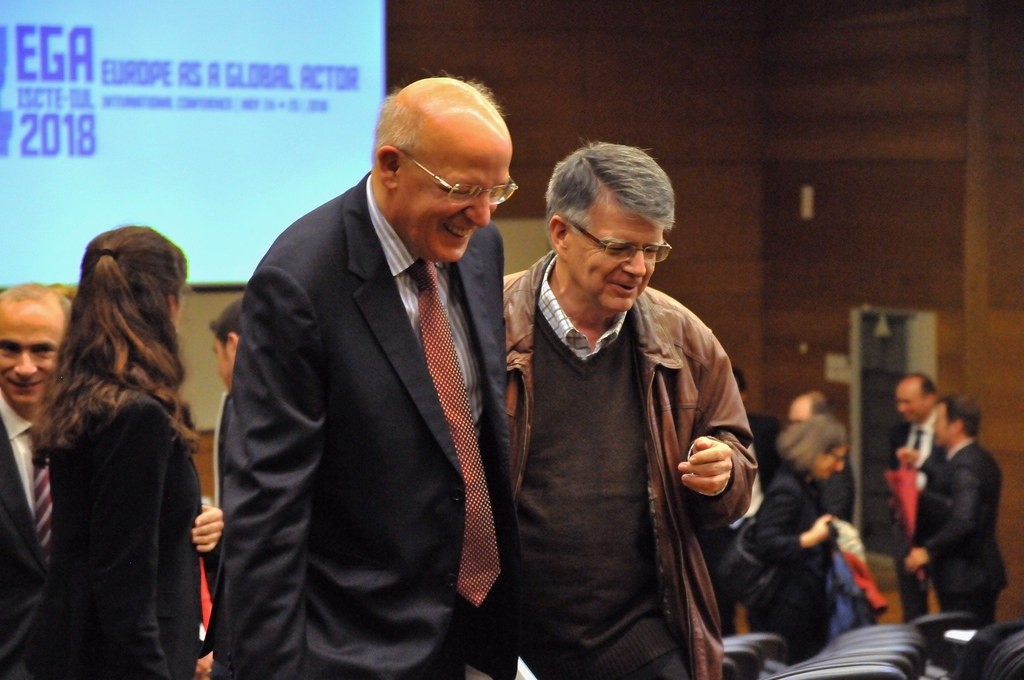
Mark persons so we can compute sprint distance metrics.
[0,77,756,680]
[697,372,1009,665]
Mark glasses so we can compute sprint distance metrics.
[393,145,520,207]
[831,452,845,462]
[568,219,673,263]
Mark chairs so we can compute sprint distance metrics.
[719,613,1024,680]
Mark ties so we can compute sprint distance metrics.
[914,430,924,449]
[412,258,502,608]
[33,452,51,557]
